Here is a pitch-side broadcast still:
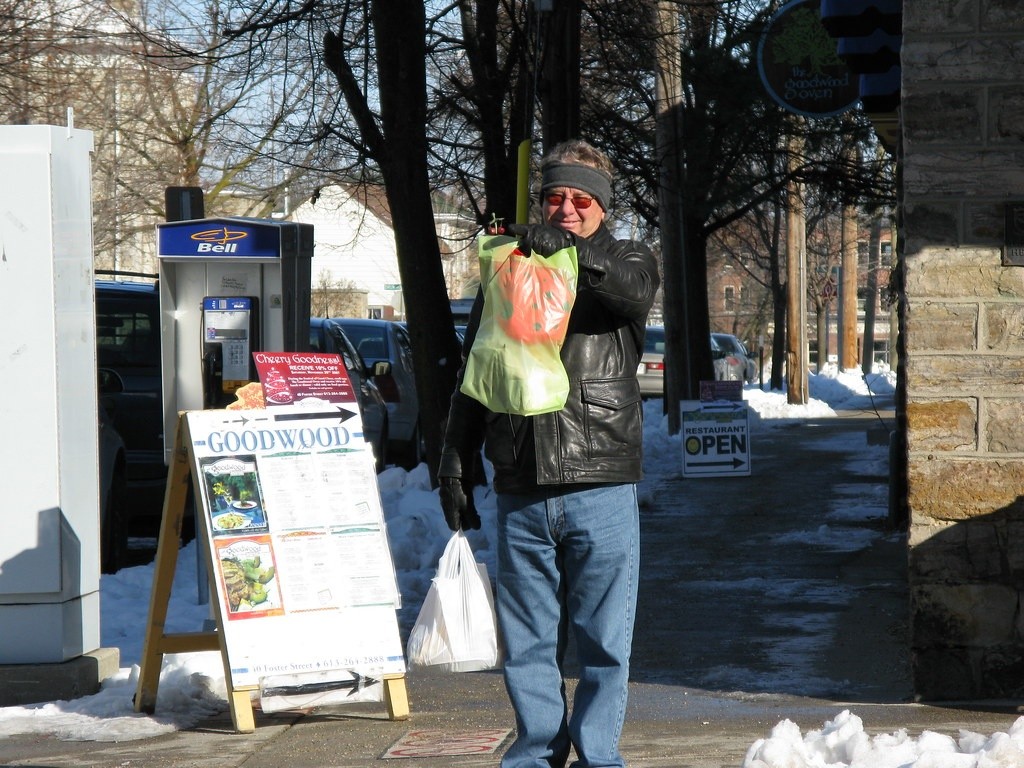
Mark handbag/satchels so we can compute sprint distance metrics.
[460,234,579,417]
[406,522,507,675]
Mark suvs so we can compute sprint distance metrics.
[95,270,236,575]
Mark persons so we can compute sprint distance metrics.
[440,137,661,768]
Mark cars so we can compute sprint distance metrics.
[330,317,423,471]
[637,324,729,403]
[391,318,469,350]
[712,332,760,384]
[310,316,389,475]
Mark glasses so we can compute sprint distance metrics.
[544,191,596,209]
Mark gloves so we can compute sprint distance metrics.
[438,477,481,532]
[508,224,574,258]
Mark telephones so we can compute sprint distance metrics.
[199,296,259,409]
